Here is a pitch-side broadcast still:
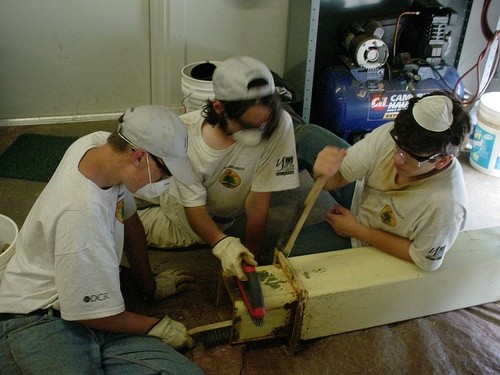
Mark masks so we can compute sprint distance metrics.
[135,154,172,198]
[230,123,267,145]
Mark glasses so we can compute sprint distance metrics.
[149,153,173,178]
[229,111,272,129]
[389,127,443,168]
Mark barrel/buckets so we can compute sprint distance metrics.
[469,91,500,178]
[180,61,219,113]
[0,213,20,276]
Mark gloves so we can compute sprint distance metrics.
[147,315,195,349]
[212,235,258,281]
[150,269,195,298]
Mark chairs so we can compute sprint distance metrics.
[297,124,357,211]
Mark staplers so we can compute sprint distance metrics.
[233,265,264,321]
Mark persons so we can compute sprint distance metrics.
[0,106,210,375]
[132,56,300,282]
[259,89,474,271]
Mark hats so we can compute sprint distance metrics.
[215,56,275,100]
[117,105,199,184]
